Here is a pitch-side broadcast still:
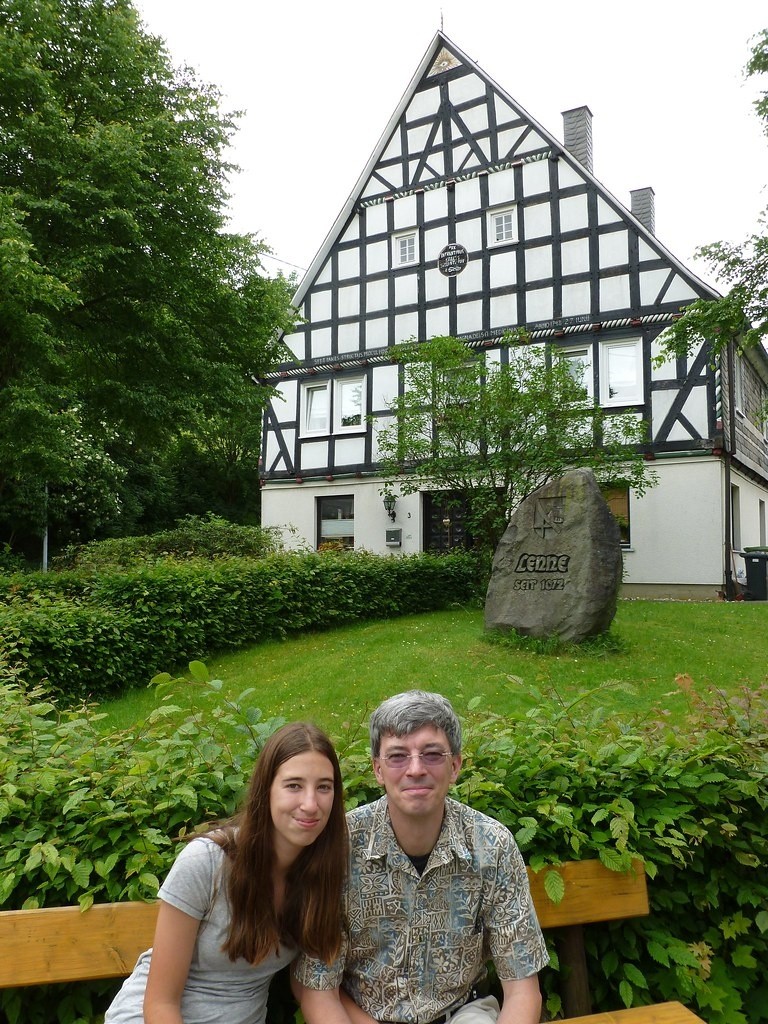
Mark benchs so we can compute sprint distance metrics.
[0,857,707,1024]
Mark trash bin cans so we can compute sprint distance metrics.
[743,546,768,553]
[739,551,768,601]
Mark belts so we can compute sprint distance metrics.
[379,989,477,1024]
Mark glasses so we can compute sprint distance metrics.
[377,748,455,769]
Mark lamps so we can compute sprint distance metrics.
[383,494,396,523]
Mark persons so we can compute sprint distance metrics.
[105,722,380,1024]
[290,690,550,1024]
